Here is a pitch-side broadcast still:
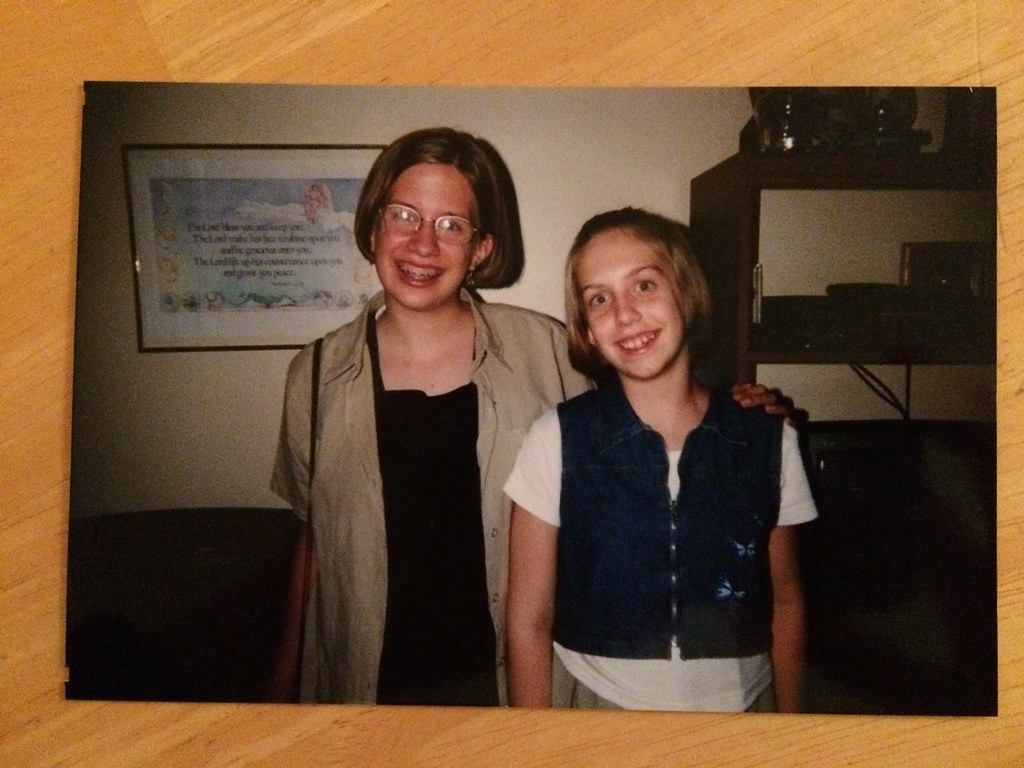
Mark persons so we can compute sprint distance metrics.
[504,206,821,713]
[272,126,800,709]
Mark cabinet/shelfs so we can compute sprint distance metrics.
[688,149,997,717]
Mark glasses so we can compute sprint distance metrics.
[378,204,481,246]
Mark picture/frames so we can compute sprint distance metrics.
[121,140,387,352]
